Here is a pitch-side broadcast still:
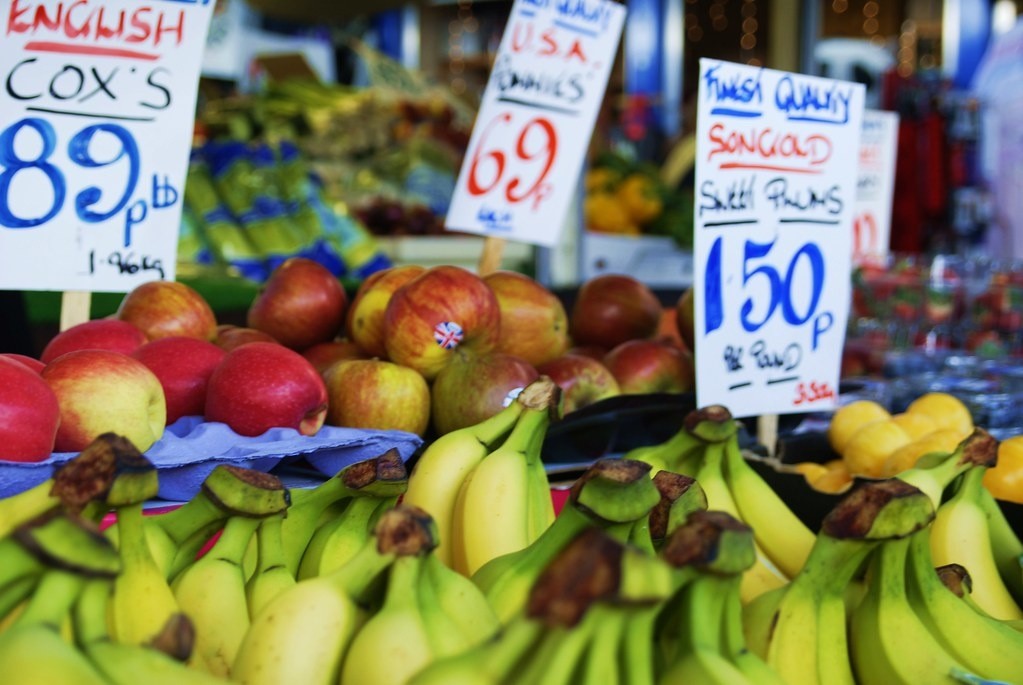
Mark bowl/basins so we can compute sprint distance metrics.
[804,253,1023,432]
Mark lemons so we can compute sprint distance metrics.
[786,394,1023,504]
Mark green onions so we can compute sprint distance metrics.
[200,74,387,156]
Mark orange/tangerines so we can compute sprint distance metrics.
[583,166,664,235]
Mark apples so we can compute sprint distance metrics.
[0,256,704,465]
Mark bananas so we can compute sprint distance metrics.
[886,428,1023,633]
[0,377,828,685]
[735,474,1023,685]
